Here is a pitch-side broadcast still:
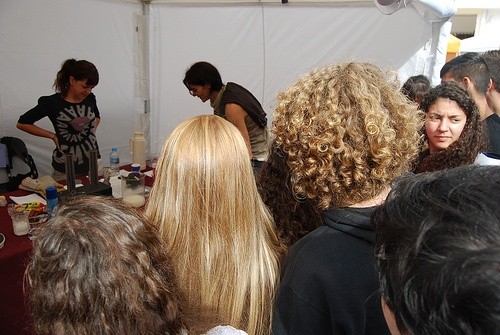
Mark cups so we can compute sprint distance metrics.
[121,172,145,207]
[11,213,30,236]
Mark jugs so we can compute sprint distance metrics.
[129,132,146,170]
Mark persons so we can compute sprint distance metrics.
[183,62,273,187]
[143,115,288,335]
[440,52,500,160]
[478,50,500,119]
[370,166,500,335]
[272,60,427,335]
[409,81,500,175]
[399,75,433,105]
[16,59,101,183]
[23,195,252,335]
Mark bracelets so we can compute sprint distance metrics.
[47,131,56,140]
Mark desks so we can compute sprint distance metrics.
[0,159,155,335]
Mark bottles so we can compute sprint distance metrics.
[109,147,120,172]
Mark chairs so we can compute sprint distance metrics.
[0,136,38,192]
